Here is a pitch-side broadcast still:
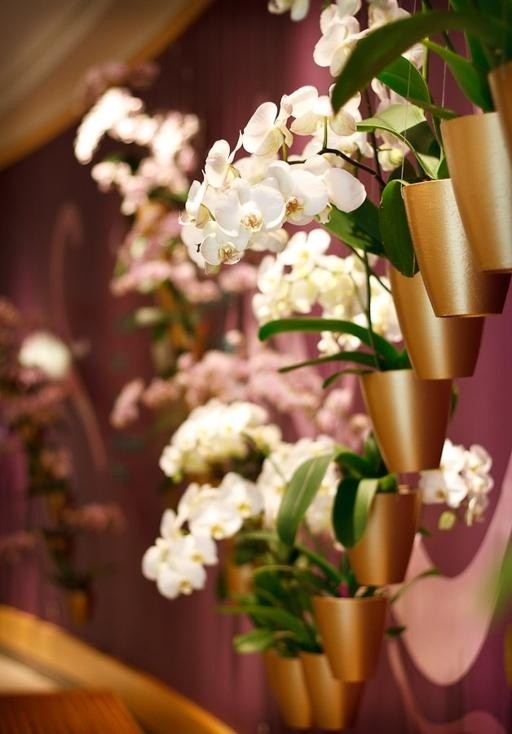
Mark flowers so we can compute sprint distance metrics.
[16,1,511,676]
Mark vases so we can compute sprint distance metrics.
[257,585,393,731]
